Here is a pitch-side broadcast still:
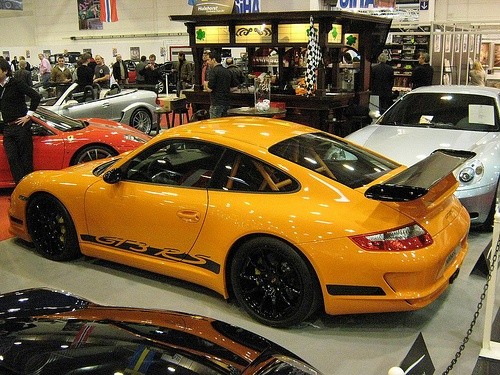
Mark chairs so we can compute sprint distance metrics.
[104,84,121,97]
[82,83,101,102]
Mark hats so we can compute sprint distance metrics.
[378,53,386,62]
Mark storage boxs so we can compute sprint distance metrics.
[159,98,186,111]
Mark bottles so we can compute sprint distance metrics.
[294,52,299,66]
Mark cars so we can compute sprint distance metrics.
[112,60,143,84]
[0,287,324,375]
[155,61,193,93]
[320,85,500,230]
[48,51,85,68]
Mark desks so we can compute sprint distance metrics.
[227,107,287,119]
[153,108,189,135]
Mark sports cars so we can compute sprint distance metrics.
[6,117,471,330]
[0,107,159,190]
[29,82,160,136]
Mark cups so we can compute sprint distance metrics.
[204,81,209,92]
[263,100,270,111]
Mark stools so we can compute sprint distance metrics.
[346,91,371,134]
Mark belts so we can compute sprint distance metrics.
[5,120,19,126]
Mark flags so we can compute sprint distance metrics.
[100,0,118,22]
[304,15,323,98]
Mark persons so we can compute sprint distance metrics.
[0,56,41,185]
[203,51,245,118]
[176,51,192,97]
[411,53,433,91]
[467,62,485,87]
[369,53,394,115]
[0,51,159,92]
